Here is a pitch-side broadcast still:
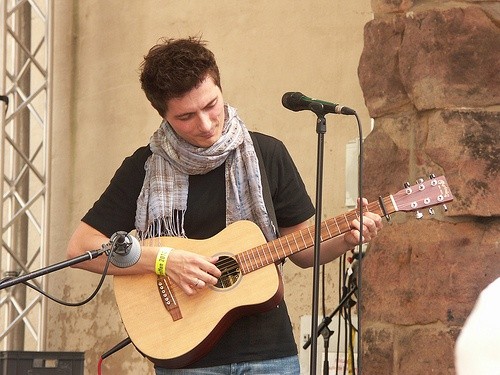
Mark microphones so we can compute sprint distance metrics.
[109,232,141,269]
[282,92,356,115]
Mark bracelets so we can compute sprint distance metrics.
[155,247,174,277]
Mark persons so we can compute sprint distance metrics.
[65,36,383,375]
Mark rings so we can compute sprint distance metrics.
[191,280,200,287]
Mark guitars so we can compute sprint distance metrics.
[113,175,453,369]
